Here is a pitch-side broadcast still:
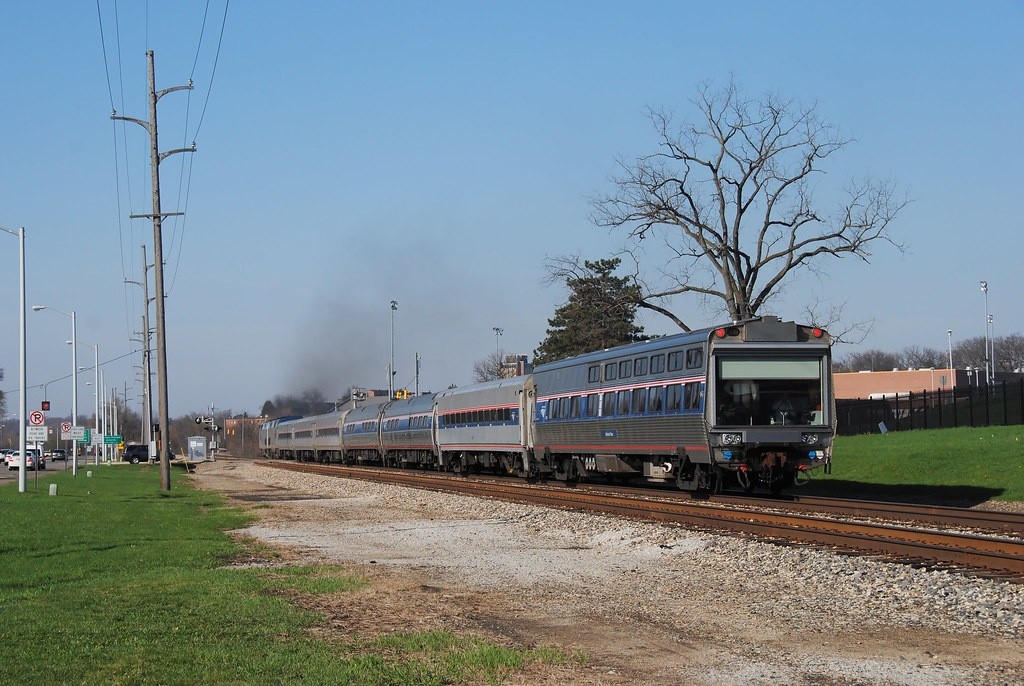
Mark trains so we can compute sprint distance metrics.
[257,315,839,502]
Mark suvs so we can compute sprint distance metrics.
[123,445,176,464]
[51,449,69,463]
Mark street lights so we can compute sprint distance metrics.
[492,327,504,380]
[32,305,79,476]
[981,281,996,385]
[390,300,398,398]
[947,329,954,389]
[67,340,118,466]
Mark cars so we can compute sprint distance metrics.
[0,449,40,471]
[44,451,51,457]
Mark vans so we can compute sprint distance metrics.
[26,444,48,469]
